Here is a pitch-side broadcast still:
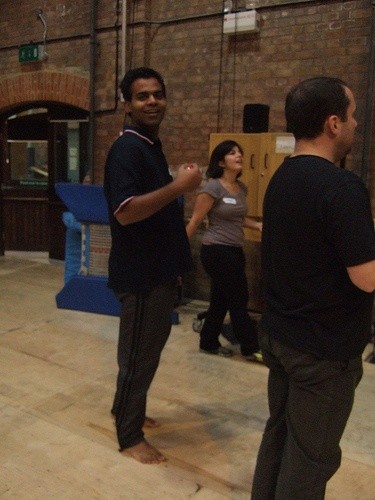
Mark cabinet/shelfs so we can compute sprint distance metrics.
[204,131,296,242]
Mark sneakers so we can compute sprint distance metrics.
[240,349,264,363]
[199,344,233,357]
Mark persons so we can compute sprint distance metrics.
[251,74,375,500]
[104,67,205,466]
[185,140,263,364]
